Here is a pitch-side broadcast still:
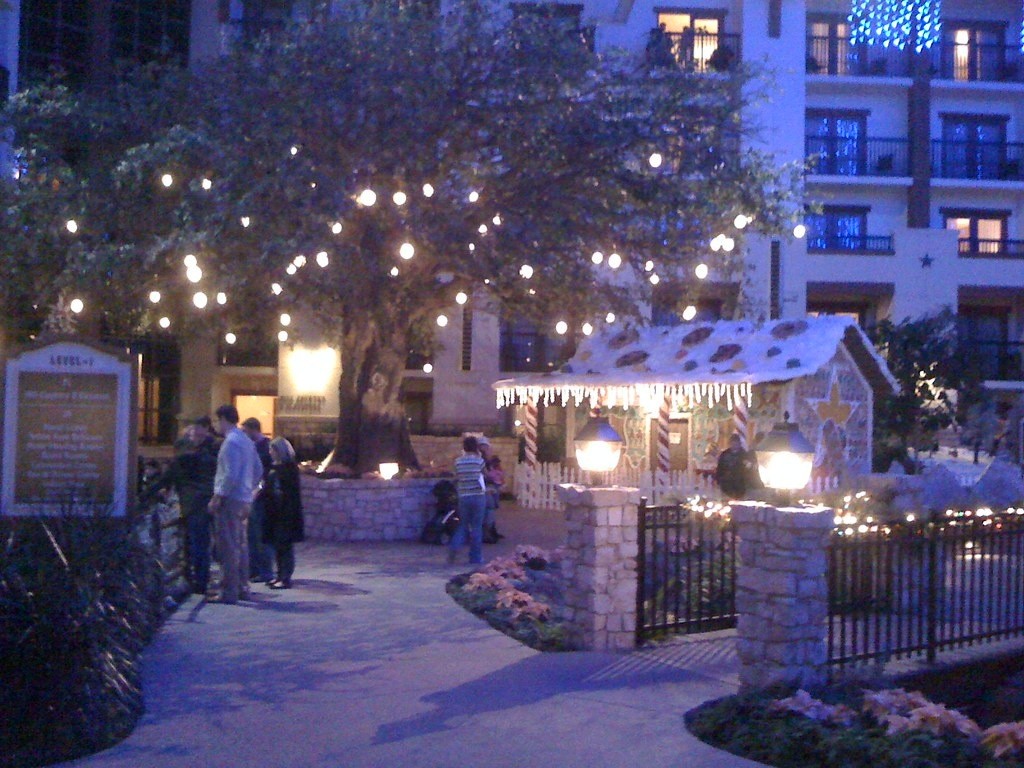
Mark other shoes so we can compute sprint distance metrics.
[207,594,236,604]
[238,591,250,600]
[482,526,504,544]
[249,574,271,582]
[470,557,484,565]
[447,547,455,564]
[266,576,291,589]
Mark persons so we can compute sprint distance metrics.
[448,436,487,565]
[746,431,764,490]
[248,434,305,589]
[716,434,753,505]
[474,432,503,542]
[240,419,274,582]
[138,413,223,596]
[428,480,456,539]
[207,405,263,606]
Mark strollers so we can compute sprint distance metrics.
[422,478,459,545]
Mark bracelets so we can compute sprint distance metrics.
[257,480,264,489]
[210,494,221,500]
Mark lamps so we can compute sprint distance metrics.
[751,412,817,508]
[379,461,398,480]
[572,408,625,490]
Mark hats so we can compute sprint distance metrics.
[476,436,489,447]
